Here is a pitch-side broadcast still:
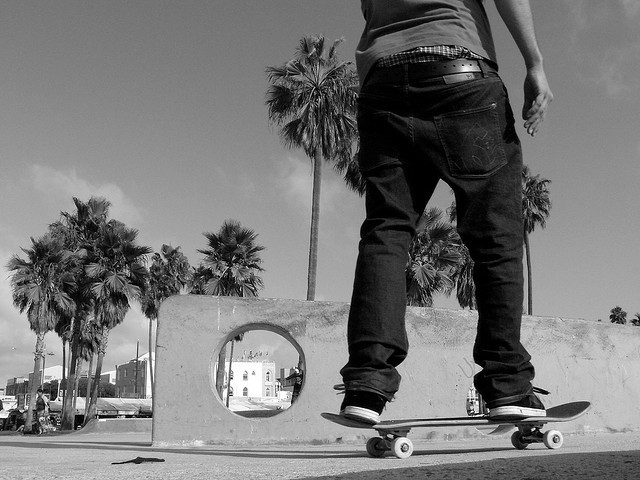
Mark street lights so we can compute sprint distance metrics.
[32,350,54,392]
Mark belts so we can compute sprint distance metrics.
[364,60,498,84]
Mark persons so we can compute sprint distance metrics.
[35,389,50,435]
[334,1,554,425]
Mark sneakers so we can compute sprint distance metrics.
[488,387,550,419]
[333,382,386,425]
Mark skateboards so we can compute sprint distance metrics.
[321,400,589,458]
